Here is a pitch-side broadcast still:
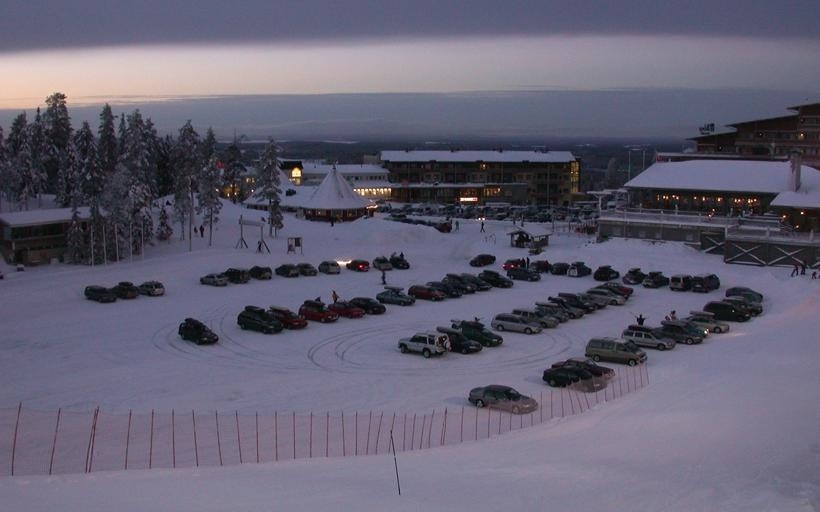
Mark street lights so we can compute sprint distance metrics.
[627,149,641,206]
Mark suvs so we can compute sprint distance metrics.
[433,220,453,233]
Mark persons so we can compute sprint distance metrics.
[200,225,204,238]
[332,291,339,304]
[400,252,404,258]
[455,220,459,232]
[791,263,817,279]
[382,269,386,285]
[670,309,679,321]
[480,222,486,233]
[521,256,530,269]
[637,314,646,325]
